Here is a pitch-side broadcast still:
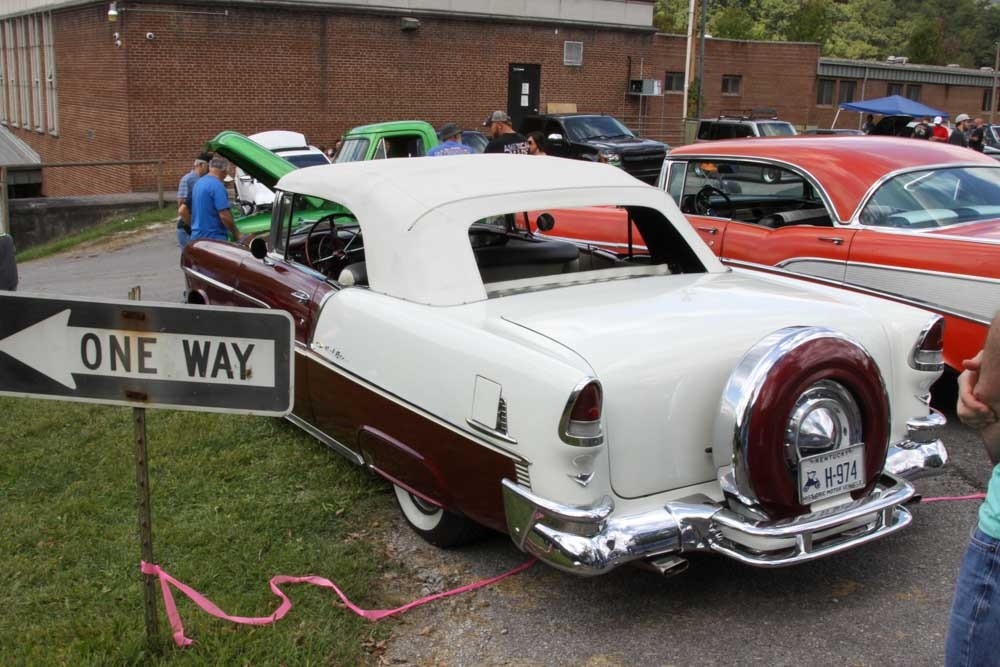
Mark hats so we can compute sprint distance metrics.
[440,122,461,141]
[955,113,974,125]
[481,110,507,127]
[197,153,212,165]
[933,116,942,124]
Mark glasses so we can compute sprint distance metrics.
[529,140,536,144]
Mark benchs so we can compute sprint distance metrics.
[475,242,580,286]
[875,205,1000,228]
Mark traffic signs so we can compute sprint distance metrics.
[0,294,296,416]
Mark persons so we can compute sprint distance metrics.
[863,114,875,130]
[481,111,530,154]
[912,117,937,140]
[191,158,244,241]
[527,131,547,155]
[949,113,974,148]
[931,116,948,142]
[945,309,1000,667]
[425,122,475,157]
[177,153,213,250]
[969,118,985,152]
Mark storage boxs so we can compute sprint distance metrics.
[684,77,703,143]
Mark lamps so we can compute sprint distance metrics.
[106,3,228,22]
[401,18,420,31]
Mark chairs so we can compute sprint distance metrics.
[338,260,369,286]
[768,208,832,229]
[858,205,906,225]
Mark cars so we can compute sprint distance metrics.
[180,110,1000,575]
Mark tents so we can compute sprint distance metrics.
[831,95,950,129]
